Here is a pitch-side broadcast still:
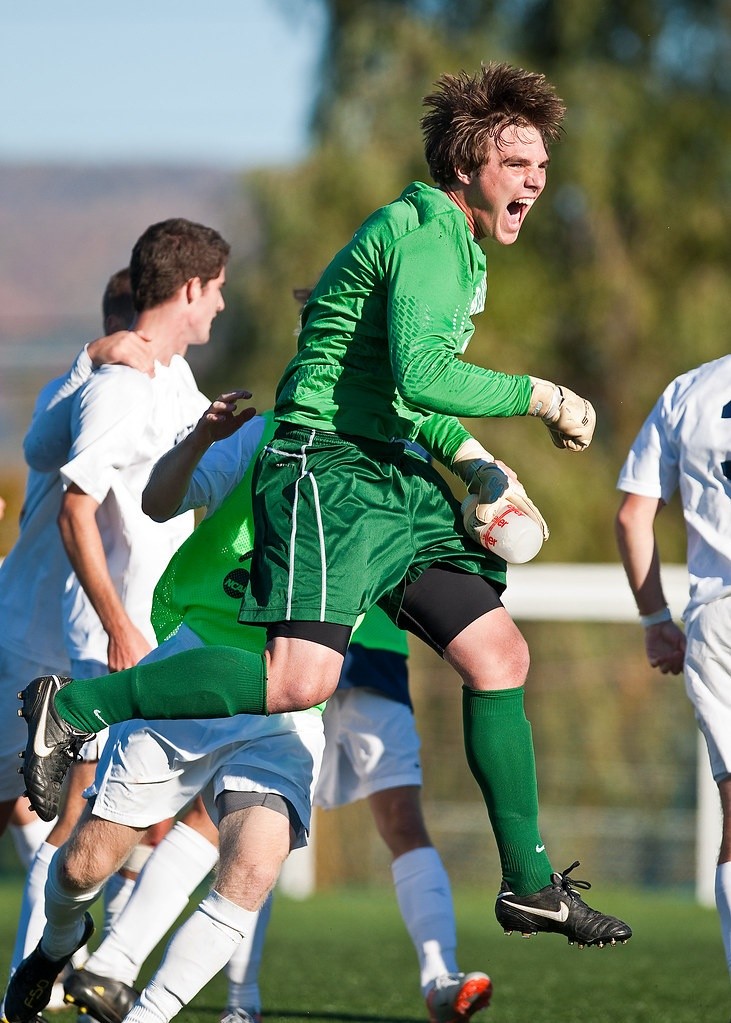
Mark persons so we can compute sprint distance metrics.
[0,219,494,1023]
[16,63,631,949]
[615,354,731,971]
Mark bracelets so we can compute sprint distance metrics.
[639,606,671,630]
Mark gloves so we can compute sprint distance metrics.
[467,472,550,556]
[525,380,598,454]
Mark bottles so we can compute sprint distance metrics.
[459,492,544,565]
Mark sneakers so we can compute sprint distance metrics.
[17,673,94,826]
[424,972,494,1023]
[62,966,142,1023]
[217,1004,263,1023]
[3,909,96,1023]
[492,860,634,948]
